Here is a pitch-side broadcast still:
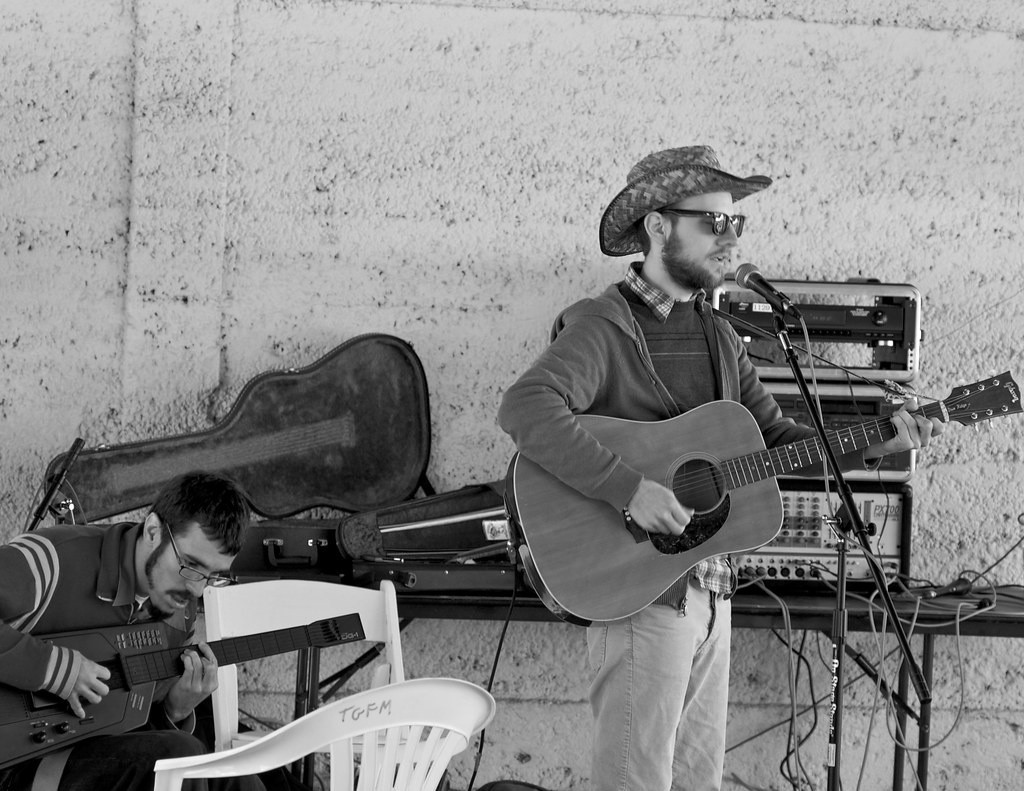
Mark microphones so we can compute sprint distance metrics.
[922,579,972,599]
[735,263,803,318]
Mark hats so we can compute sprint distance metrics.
[597,143,772,257]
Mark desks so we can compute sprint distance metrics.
[197,563,1024,791]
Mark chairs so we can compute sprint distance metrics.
[203,580,412,755]
[152,677,498,791]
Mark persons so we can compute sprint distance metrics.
[0,470,250,791]
[498,147,945,791]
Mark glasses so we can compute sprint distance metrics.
[162,513,241,588]
[654,205,748,239]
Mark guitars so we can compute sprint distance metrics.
[504,369,1024,628]
[0,611,366,770]
